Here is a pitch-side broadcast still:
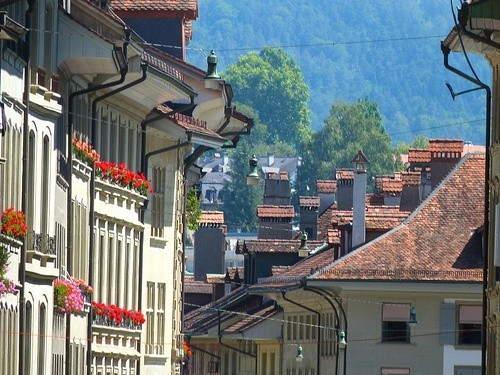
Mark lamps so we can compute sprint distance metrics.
[246,154,259,185]
[296,233,311,257]
[408,306,417,327]
[295,344,304,362]
[203,49,221,89]
[338,329,347,349]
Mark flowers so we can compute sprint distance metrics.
[54,277,146,330]
[182,342,192,355]
[0,265,16,297]
[0,207,28,240]
[72,137,155,198]
